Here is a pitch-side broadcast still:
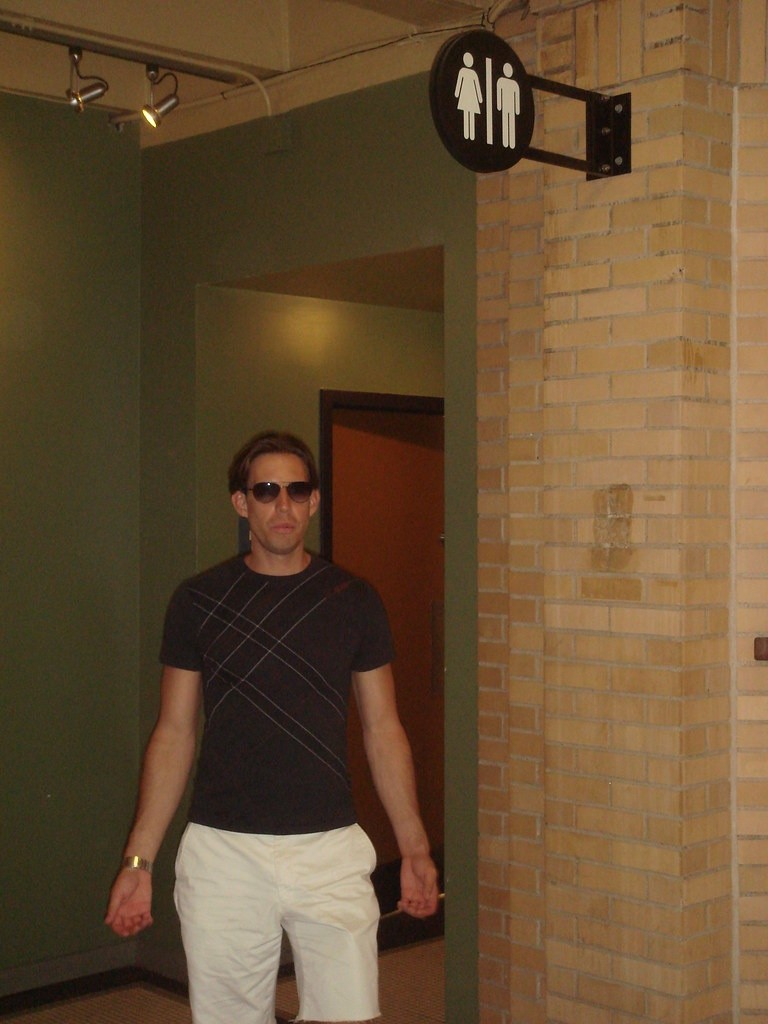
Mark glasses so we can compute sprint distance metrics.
[243,481,316,504]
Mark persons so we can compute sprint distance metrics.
[106,433,437,1024]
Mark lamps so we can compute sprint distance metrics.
[140,64,180,128]
[64,47,110,116]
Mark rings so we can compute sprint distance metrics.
[408,900,417,906]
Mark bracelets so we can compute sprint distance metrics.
[119,856,154,874]
